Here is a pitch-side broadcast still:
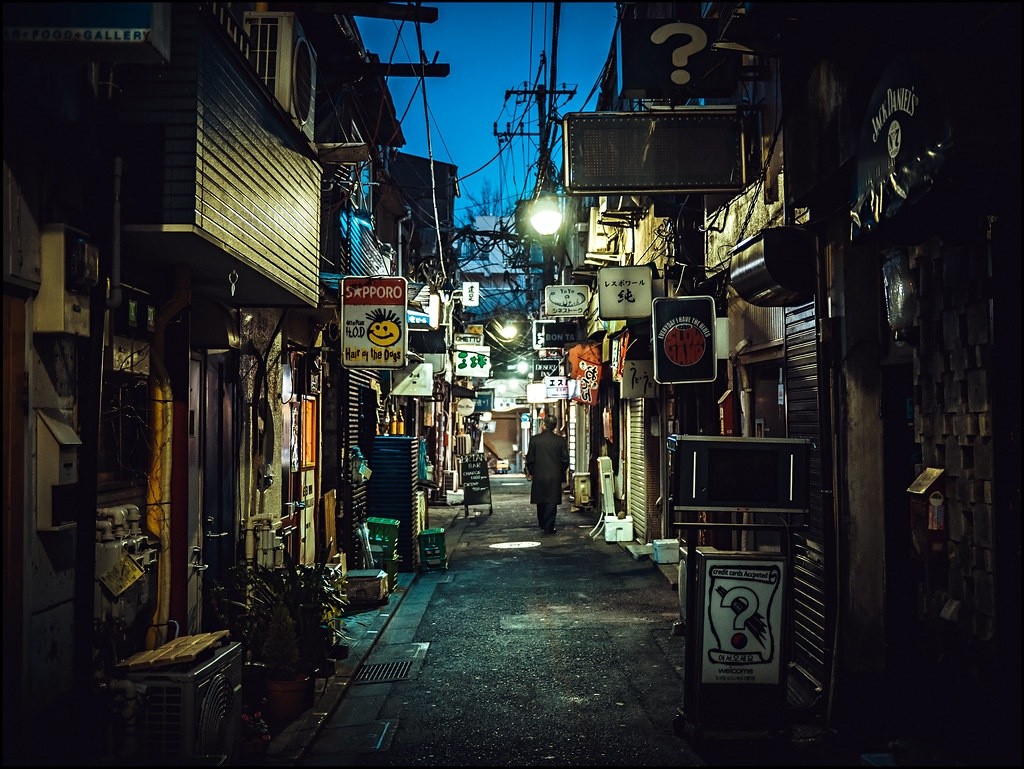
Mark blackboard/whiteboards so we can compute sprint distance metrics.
[462,461,492,505]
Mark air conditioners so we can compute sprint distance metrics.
[127,641,244,769]
[444,470,458,494]
[457,435,471,456]
[242,11,319,141]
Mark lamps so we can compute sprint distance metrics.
[530,192,568,235]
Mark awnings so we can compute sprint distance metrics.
[389,150,460,279]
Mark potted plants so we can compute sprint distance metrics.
[209,541,348,720]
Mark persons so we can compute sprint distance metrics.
[526,415,570,534]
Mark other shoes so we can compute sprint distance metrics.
[545,528,557,534]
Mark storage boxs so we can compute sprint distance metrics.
[345,517,449,600]
